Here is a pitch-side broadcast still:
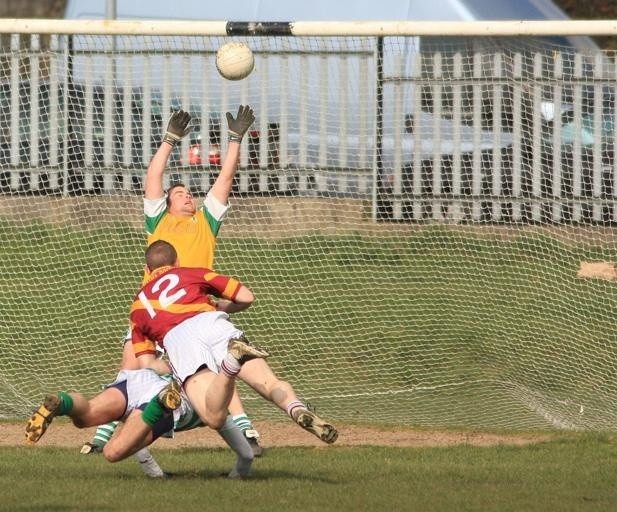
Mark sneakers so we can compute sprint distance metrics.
[158,380,183,410]
[227,338,270,366]
[290,402,339,444]
[24,393,63,446]
[243,429,263,457]
[138,454,164,478]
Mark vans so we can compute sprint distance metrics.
[53,0,615,201]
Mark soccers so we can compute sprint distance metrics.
[216,43,253,79]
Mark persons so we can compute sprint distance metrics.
[130,240,339,444]
[79,105,264,457]
[25,356,254,480]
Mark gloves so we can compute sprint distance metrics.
[226,105,255,144]
[163,109,191,146]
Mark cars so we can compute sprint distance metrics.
[375,99,615,225]
[1,78,281,201]
